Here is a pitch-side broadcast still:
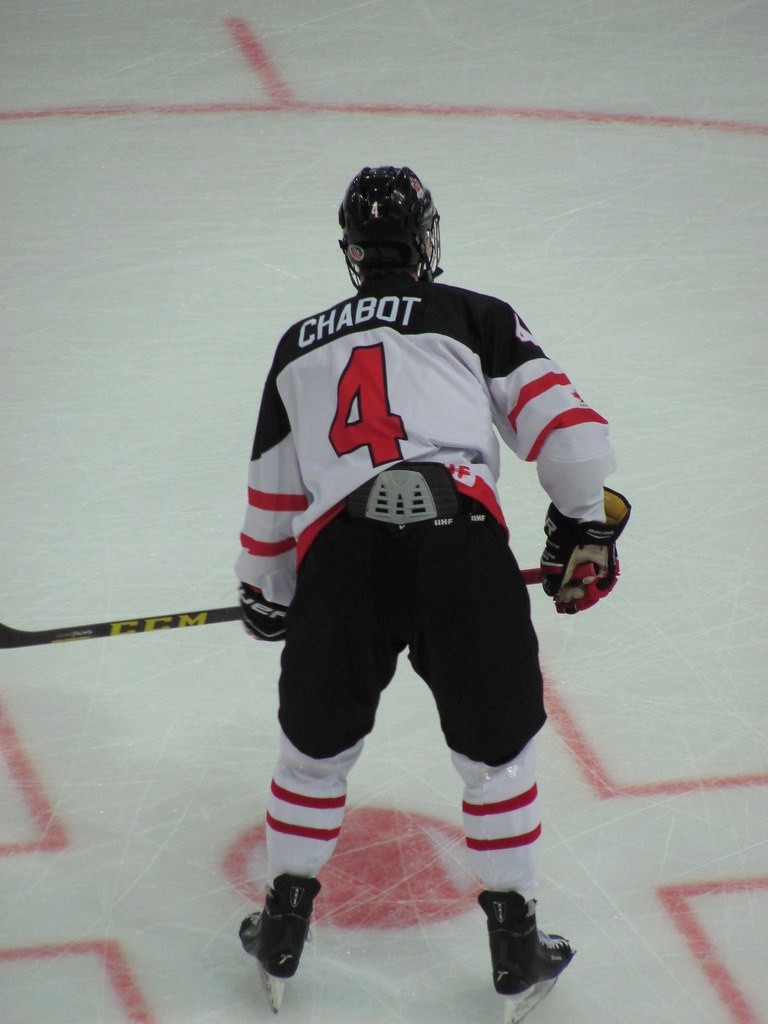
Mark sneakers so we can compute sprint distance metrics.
[239,873,320,1014]
[477,891,572,1024]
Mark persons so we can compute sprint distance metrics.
[236,167,620,996]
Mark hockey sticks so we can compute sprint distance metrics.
[0,560,599,651]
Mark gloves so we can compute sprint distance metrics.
[239,584,290,642]
[539,487,632,614]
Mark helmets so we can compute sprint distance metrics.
[338,166,442,289]
